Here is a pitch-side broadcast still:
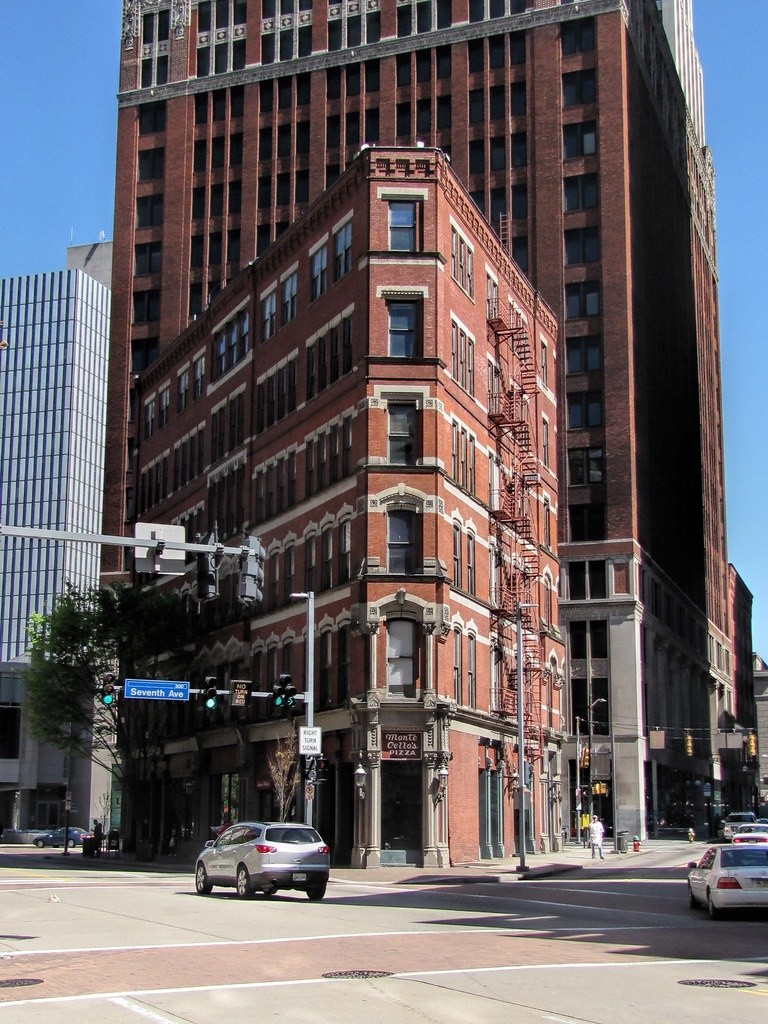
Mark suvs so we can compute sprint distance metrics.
[195,820,331,900]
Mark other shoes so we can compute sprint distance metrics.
[601,857,604,859]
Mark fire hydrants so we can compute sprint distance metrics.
[632,835,641,852]
[687,828,695,843]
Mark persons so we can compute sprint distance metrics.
[91,819,103,858]
[216,816,234,837]
[589,815,605,860]
[167,822,177,856]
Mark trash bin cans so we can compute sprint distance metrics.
[106,831,118,850]
[617,830,629,854]
[82,838,98,857]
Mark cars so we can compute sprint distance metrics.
[33,826,95,848]
[754,818,768,824]
[731,823,768,843]
[687,842,768,920]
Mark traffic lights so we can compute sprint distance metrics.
[102,673,115,705]
[206,678,218,709]
[317,758,329,782]
[274,686,285,712]
[283,675,297,708]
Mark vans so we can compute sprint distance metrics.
[721,811,756,843]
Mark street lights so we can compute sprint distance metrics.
[515,600,539,872]
[289,591,314,827]
[62,799,71,856]
[588,698,607,821]
